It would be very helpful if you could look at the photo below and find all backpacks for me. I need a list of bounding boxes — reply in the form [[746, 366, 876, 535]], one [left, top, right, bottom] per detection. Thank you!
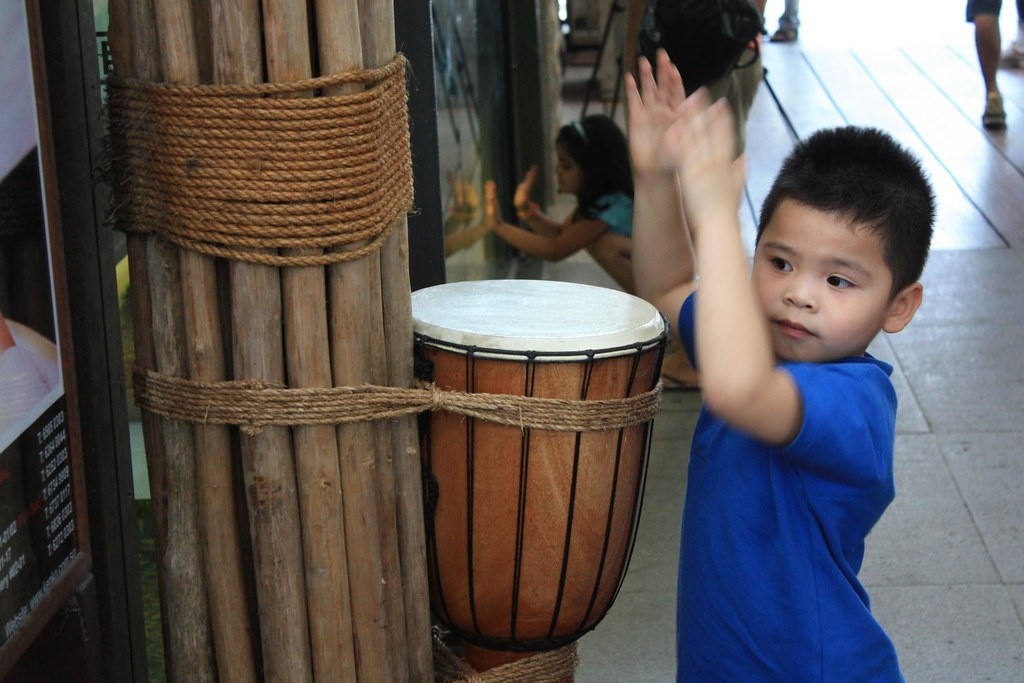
[[638, 0, 768, 97]]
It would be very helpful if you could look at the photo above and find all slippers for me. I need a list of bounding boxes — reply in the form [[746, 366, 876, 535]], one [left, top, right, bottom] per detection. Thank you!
[[660, 372, 700, 393]]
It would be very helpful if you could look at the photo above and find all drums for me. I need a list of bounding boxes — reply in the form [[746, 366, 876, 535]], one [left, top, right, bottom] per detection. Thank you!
[[415, 276, 670, 683]]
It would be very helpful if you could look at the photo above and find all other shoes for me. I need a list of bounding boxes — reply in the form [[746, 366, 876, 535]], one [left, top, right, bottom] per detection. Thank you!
[[998, 41, 1024, 70], [982, 91, 1007, 128], [770, 28, 798, 42]]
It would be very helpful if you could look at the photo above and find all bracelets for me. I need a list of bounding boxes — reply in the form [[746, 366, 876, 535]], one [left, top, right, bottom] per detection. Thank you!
[[517, 201, 538, 221]]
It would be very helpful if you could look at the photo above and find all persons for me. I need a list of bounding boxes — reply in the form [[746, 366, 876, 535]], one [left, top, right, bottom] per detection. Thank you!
[[755, 0, 800, 42], [486, 114, 636, 295], [443, 170, 486, 258], [639, 0, 764, 390], [966, 0, 1024, 127], [625, 49, 937, 683]]
